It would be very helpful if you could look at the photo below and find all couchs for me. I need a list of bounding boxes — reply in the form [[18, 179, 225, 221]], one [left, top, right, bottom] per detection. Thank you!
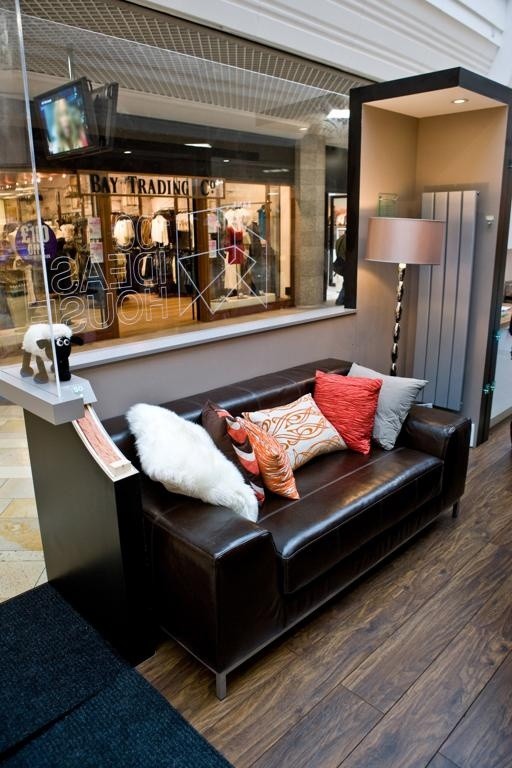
[[100, 355, 472, 700]]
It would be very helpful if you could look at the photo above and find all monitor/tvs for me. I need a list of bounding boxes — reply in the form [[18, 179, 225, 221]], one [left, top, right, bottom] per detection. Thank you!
[[91, 81, 120, 153], [32, 73, 103, 162]]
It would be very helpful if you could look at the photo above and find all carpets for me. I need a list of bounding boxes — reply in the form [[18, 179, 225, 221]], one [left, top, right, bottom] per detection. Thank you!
[[1, 584, 236, 767]]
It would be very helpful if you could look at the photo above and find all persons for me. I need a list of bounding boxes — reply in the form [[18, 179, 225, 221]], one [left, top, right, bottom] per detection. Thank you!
[[334, 229, 347, 304], [50, 98, 85, 152], [225, 214, 246, 265]]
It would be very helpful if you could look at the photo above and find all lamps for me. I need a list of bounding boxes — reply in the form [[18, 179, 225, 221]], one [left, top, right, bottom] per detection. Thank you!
[[363, 217, 448, 376]]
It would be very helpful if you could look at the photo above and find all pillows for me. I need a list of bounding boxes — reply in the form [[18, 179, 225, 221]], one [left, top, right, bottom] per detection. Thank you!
[[309, 367, 385, 457], [125, 400, 264, 524], [238, 391, 350, 474], [346, 361, 429, 454], [188, 397, 267, 507], [233, 414, 302, 502]]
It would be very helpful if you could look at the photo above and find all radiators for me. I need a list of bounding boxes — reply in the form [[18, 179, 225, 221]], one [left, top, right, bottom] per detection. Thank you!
[[412, 189, 479, 413]]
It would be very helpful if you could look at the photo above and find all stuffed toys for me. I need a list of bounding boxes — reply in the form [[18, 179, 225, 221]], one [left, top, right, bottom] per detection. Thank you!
[[18, 322, 87, 385]]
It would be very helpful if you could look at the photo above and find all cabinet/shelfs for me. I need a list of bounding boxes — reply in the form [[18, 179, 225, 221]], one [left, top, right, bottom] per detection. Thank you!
[[489, 326, 512, 430]]
